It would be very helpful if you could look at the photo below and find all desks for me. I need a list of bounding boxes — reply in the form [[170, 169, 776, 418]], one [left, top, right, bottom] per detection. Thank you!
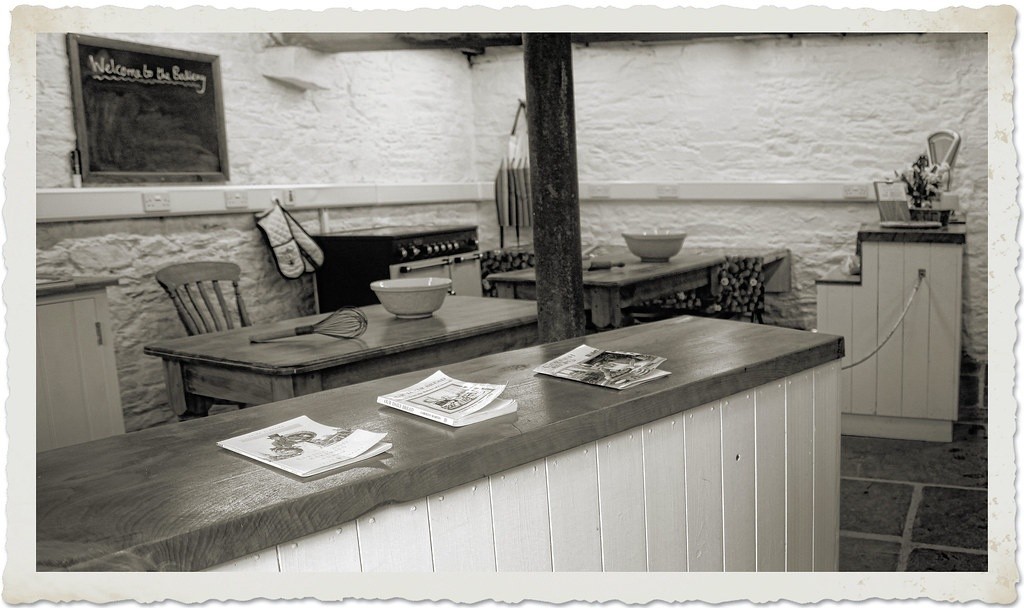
[[144, 296, 538, 422], [486, 247, 792, 341]]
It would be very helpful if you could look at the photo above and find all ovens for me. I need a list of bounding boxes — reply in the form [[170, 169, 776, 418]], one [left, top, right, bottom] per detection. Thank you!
[[310, 224, 484, 311]]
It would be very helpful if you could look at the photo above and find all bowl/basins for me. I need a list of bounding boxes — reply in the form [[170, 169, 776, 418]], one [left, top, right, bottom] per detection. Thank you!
[[619, 232, 687, 263], [370, 277, 452, 319]]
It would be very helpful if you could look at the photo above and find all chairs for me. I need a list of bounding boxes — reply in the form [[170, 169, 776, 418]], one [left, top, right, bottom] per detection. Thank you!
[[155, 262, 252, 338]]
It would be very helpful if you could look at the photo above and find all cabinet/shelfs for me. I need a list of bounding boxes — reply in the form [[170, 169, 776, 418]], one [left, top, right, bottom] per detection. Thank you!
[[36, 273, 125, 453], [816, 218, 968, 443]]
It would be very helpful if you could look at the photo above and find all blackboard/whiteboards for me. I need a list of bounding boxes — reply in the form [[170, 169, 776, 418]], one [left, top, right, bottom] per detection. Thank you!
[[65, 32, 232, 184]]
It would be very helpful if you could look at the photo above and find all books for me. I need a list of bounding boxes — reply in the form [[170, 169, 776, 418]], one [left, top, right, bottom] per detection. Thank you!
[[533, 345, 671, 390], [215, 415, 393, 478], [377, 370, 518, 427]]
